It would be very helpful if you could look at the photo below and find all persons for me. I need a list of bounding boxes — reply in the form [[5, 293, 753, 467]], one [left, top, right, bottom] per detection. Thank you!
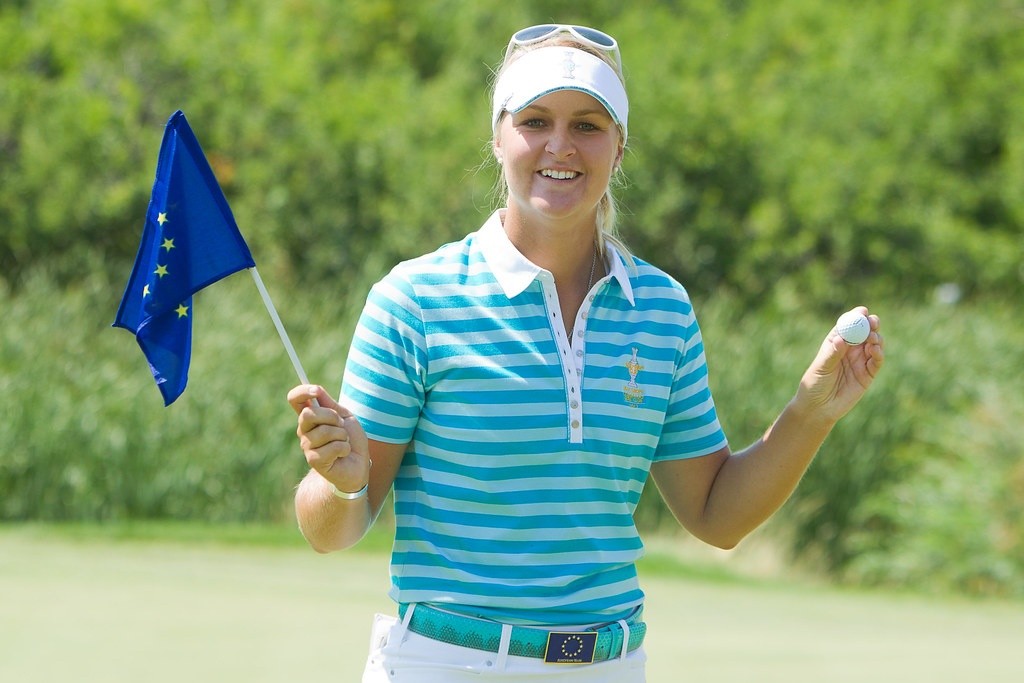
[[288, 25, 884, 683]]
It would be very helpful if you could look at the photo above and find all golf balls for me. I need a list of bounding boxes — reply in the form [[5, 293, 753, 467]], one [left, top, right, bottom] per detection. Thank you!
[[836, 311, 871, 345]]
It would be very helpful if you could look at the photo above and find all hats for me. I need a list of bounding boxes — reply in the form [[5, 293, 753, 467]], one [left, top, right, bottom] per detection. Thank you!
[[491, 46, 629, 148]]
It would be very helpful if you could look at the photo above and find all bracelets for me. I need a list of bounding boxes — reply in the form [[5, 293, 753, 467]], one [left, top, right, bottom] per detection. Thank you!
[[329, 483, 369, 500]]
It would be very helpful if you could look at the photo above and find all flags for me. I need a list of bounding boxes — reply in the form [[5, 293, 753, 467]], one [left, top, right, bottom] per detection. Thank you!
[[111, 111, 255, 407]]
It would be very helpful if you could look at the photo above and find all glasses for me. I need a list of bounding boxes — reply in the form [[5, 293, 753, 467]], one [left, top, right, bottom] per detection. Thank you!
[[502, 24, 622, 76]]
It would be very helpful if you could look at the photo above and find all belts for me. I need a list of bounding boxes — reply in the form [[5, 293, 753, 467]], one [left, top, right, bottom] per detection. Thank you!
[[398, 602, 647, 665]]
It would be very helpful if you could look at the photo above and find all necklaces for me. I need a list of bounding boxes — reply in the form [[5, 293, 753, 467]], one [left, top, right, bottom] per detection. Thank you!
[[567, 242, 596, 341]]
